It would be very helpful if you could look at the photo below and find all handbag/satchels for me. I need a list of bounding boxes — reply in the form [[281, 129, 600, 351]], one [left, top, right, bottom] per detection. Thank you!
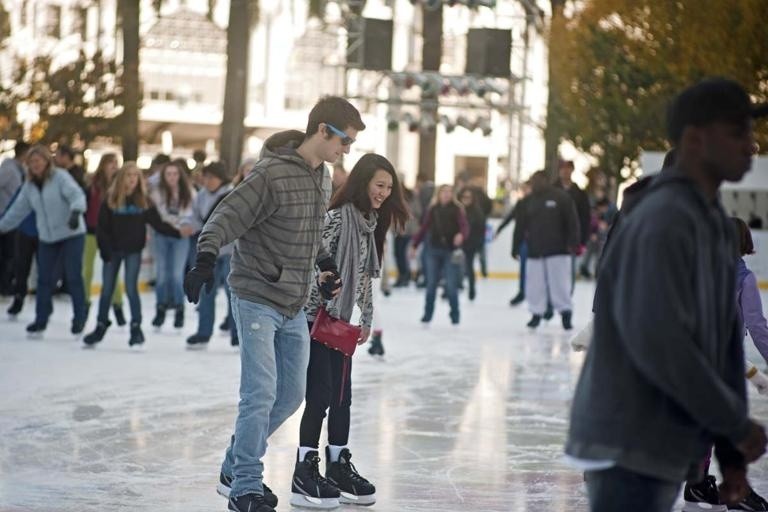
[[311, 306, 360, 356]]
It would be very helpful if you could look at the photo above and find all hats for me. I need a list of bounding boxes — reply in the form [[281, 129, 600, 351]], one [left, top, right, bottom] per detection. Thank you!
[[667, 79, 768, 122]]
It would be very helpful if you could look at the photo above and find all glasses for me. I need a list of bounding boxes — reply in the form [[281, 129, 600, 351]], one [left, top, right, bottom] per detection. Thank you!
[[327, 124, 353, 145]]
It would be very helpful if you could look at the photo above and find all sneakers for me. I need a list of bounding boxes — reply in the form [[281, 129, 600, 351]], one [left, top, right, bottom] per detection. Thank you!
[[7, 296, 209, 346], [220, 446, 375, 512], [685, 475, 768, 512]]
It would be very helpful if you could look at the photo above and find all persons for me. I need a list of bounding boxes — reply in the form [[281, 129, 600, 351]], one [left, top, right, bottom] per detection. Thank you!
[[332, 163, 349, 199], [290, 153, 413, 499], [683, 217, 768, 512], [368, 161, 616, 354], [562, 78, 768, 512], [0, 142, 258, 346], [184, 98, 367, 512]]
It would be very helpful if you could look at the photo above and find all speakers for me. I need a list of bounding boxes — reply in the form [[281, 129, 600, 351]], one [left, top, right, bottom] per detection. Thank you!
[[347, 17, 393, 71], [465, 28, 512, 78]]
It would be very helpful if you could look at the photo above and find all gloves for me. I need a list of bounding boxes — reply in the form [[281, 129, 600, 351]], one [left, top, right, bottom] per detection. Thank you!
[[318, 258, 342, 299], [185, 252, 214, 304]]
